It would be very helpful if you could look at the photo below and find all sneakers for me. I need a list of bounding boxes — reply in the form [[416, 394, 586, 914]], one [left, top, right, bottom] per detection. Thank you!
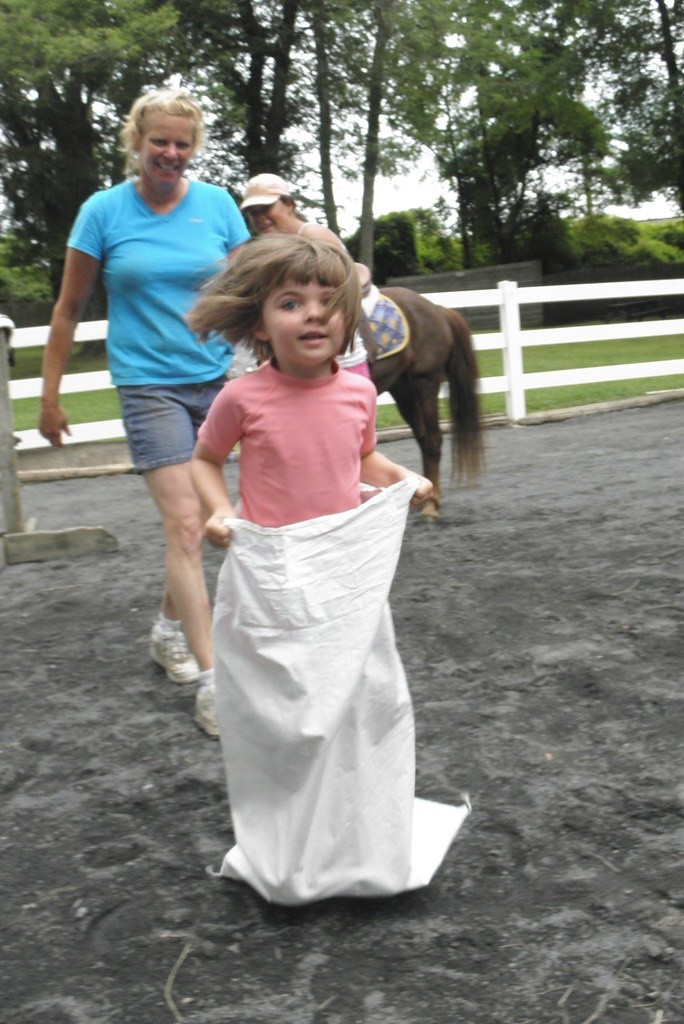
[[194, 683, 223, 740], [149, 622, 202, 684]]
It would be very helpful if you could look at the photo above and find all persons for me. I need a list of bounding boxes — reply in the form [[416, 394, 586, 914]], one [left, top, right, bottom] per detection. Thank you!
[[187, 230, 470, 903], [36, 89, 250, 735], [238, 174, 384, 504]]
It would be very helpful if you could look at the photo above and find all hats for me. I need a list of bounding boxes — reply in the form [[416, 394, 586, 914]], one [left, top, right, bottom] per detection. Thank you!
[[240, 173, 291, 210]]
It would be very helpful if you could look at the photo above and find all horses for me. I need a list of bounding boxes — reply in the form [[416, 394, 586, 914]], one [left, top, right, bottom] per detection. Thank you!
[[355, 286, 486, 529]]
